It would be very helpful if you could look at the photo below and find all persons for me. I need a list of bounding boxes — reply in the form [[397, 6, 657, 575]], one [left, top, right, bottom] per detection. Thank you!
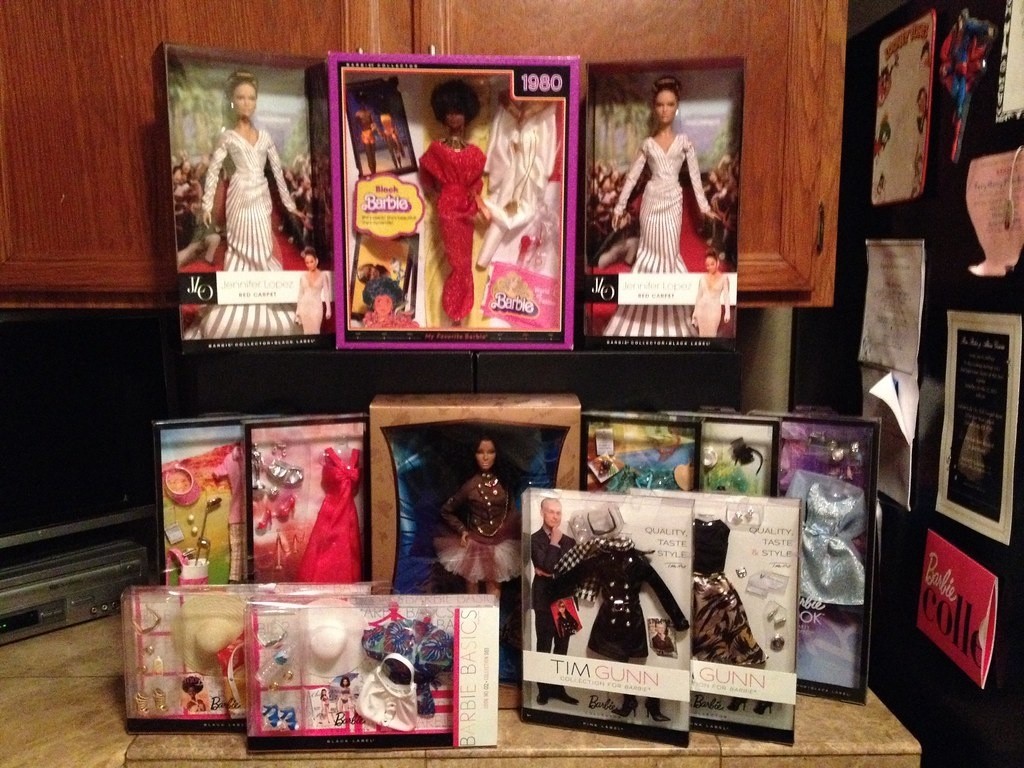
[[374, 100, 406, 172], [531, 497, 582, 705], [603, 73, 722, 336], [433, 434, 522, 606], [417, 77, 491, 329], [318, 689, 331, 725], [691, 248, 732, 339], [338, 676, 353, 713], [182, 676, 207, 713], [350, 95, 383, 175], [295, 245, 332, 336], [555, 600, 578, 638], [653, 621, 676, 653], [186, 65, 309, 339]]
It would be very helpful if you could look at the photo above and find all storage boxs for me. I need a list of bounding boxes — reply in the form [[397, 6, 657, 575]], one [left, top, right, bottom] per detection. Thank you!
[[120, 39, 886, 750]]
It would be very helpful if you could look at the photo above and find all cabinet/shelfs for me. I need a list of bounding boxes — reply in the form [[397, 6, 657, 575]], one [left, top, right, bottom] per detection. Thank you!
[[0, 0, 850, 309]]
